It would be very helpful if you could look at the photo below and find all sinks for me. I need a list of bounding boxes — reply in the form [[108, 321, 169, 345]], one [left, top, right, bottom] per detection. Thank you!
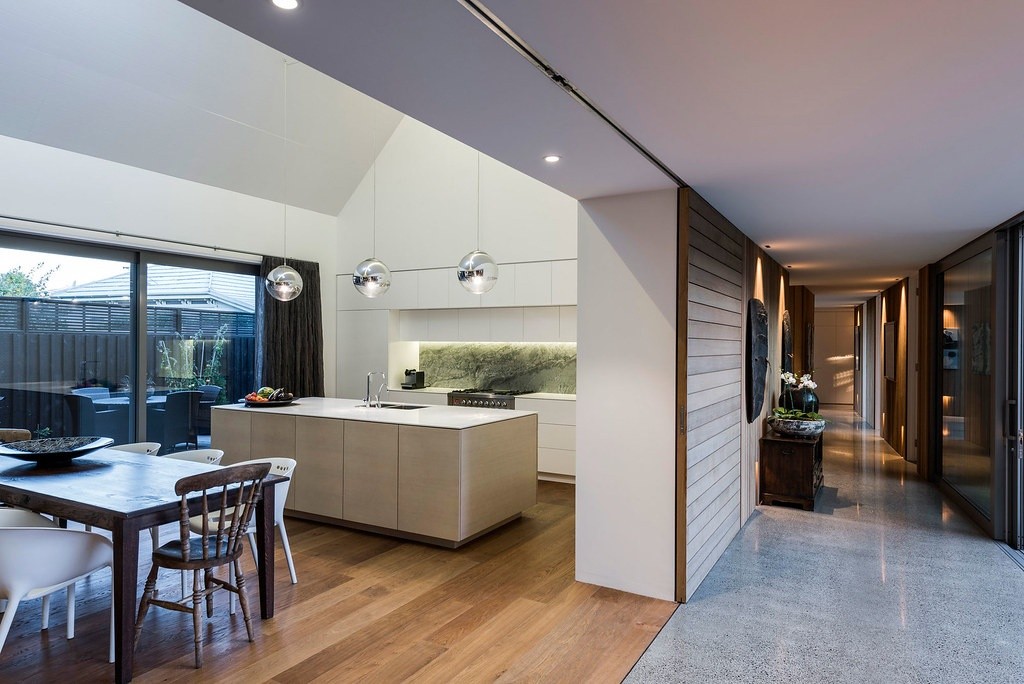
[[390, 404, 429, 410], [354, 403, 393, 409]]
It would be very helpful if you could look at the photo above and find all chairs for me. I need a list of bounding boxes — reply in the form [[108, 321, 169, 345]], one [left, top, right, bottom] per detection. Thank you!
[[72, 388, 110, 411], [147, 391, 204, 454], [64, 394, 129, 446], [0, 444, 297, 669], [196, 386, 222, 434]]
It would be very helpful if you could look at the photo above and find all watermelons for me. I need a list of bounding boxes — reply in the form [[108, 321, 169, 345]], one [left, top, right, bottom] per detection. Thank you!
[[258, 386, 275, 398]]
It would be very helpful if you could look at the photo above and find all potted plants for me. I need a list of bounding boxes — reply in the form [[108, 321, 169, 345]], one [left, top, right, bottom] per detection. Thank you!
[[767, 407, 832, 440]]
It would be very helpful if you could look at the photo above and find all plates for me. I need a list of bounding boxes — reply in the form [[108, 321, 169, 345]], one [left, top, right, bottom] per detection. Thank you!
[[0, 436, 114, 468], [238, 397, 299, 405]]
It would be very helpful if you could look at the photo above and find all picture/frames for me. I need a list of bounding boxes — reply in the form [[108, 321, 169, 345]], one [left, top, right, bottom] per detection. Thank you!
[[883, 321, 896, 381]]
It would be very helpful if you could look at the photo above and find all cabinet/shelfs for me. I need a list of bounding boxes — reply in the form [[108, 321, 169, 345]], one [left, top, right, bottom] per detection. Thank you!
[[514, 398, 577, 477], [336, 260, 578, 309], [759, 430, 825, 512]]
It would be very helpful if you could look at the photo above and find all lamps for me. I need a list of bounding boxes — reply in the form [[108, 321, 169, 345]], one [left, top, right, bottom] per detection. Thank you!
[[266, 60, 303, 302], [352, 132, 390, 297], [457, 153, 498, 295]]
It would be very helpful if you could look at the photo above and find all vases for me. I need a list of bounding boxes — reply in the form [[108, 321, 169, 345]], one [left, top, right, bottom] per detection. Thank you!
[[778, 383, 819, 417]]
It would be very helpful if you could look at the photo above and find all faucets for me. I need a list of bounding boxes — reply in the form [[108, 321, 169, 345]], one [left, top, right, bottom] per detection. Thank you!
[[363, 371, 389, 408]]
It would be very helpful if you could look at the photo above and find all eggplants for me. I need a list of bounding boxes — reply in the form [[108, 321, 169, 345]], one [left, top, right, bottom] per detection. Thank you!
[[268, 387, 294, 399]]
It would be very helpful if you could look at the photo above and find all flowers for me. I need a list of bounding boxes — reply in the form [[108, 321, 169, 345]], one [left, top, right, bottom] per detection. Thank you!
[[772, 364, 832, 424]]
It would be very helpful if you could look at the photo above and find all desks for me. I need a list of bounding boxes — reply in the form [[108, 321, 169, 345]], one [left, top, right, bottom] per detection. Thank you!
[[0, 449, 291, 684], [92, 396, 167, 409]]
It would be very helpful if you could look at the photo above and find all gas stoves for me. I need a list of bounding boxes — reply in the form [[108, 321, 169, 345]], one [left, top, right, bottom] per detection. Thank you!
[[448, 387, 534, 409]]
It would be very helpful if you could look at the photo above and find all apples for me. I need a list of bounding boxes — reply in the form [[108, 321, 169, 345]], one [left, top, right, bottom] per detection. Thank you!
[[245, 392, 270, 402]]
